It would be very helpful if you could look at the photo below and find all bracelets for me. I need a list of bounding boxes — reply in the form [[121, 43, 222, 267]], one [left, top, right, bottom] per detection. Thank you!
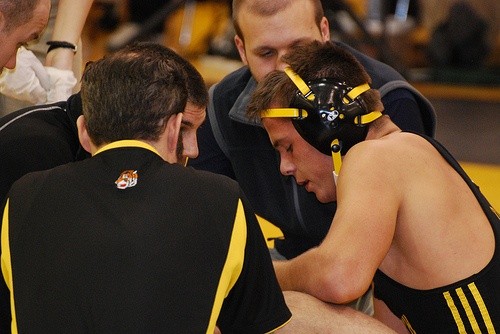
[[45, 40, 77, 52]]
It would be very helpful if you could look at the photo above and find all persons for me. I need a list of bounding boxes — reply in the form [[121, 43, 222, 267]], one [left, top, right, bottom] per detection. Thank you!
[[1, 45, 209, 199], [187, 0, 438, 259], [1, 1, 50, 72], [0, 46, 292, 334], [1, 0, 93, 105], [244, 39, 500, 334]]
